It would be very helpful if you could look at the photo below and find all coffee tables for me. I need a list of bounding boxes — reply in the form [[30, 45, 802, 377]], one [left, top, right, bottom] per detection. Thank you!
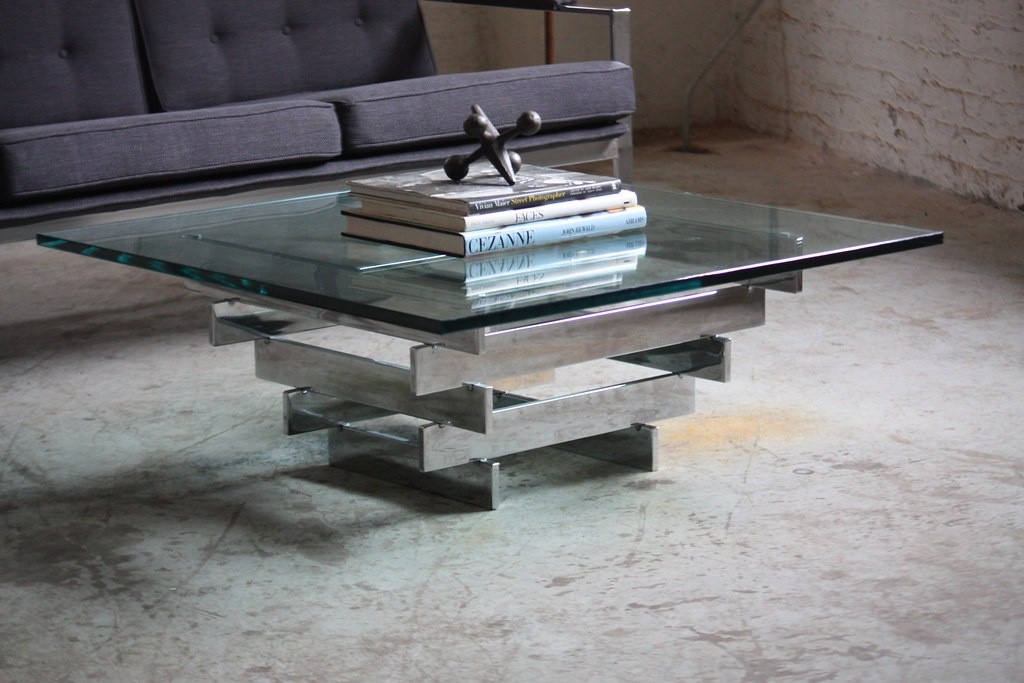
[[38, 180, 945, 511]]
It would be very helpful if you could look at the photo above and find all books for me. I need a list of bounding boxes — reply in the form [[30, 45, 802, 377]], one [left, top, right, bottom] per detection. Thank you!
[[343, 233, 646, 310], [340, 164, 646, 258]]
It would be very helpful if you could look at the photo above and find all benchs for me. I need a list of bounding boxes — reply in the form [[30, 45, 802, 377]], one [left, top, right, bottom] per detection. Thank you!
[[0, 0, 636, 245]]
